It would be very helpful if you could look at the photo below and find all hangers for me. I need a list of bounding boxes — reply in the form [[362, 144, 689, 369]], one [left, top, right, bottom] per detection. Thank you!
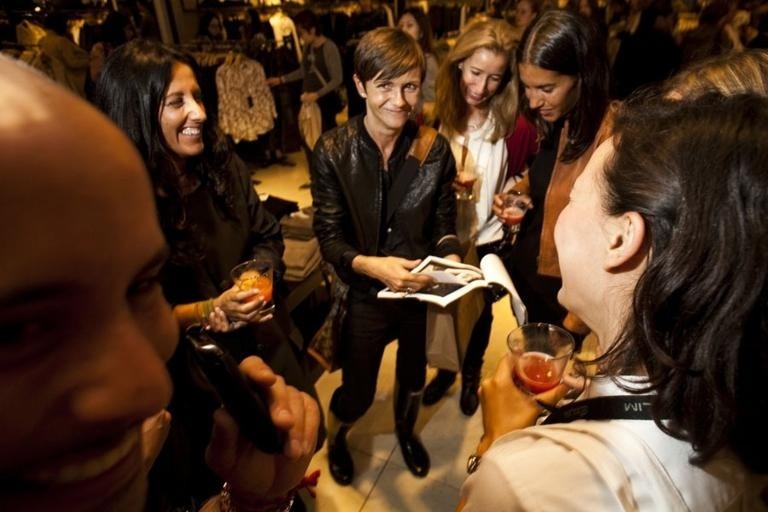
[[195, 46, 242, 67]]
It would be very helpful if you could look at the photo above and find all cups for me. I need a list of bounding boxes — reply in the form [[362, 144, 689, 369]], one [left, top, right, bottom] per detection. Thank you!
[[453, 169, 479, 201], [232, 260, 273, 312], [506, 325, 575, 392], [501, 192, 527, 226]]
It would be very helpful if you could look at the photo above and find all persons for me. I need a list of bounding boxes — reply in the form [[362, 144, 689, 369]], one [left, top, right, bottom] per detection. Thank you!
[[0, 2, 768, 512]]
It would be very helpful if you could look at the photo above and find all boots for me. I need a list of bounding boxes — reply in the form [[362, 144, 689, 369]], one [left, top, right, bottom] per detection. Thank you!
[[459, 360, 481, 417], [393, 379, 431, 479], [326, 411, 355, 486], [422, 367, 457, 406]]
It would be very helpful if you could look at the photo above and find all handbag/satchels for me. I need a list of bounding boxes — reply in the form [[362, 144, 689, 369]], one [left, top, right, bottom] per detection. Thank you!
[[304, 287, 347, 373]]
